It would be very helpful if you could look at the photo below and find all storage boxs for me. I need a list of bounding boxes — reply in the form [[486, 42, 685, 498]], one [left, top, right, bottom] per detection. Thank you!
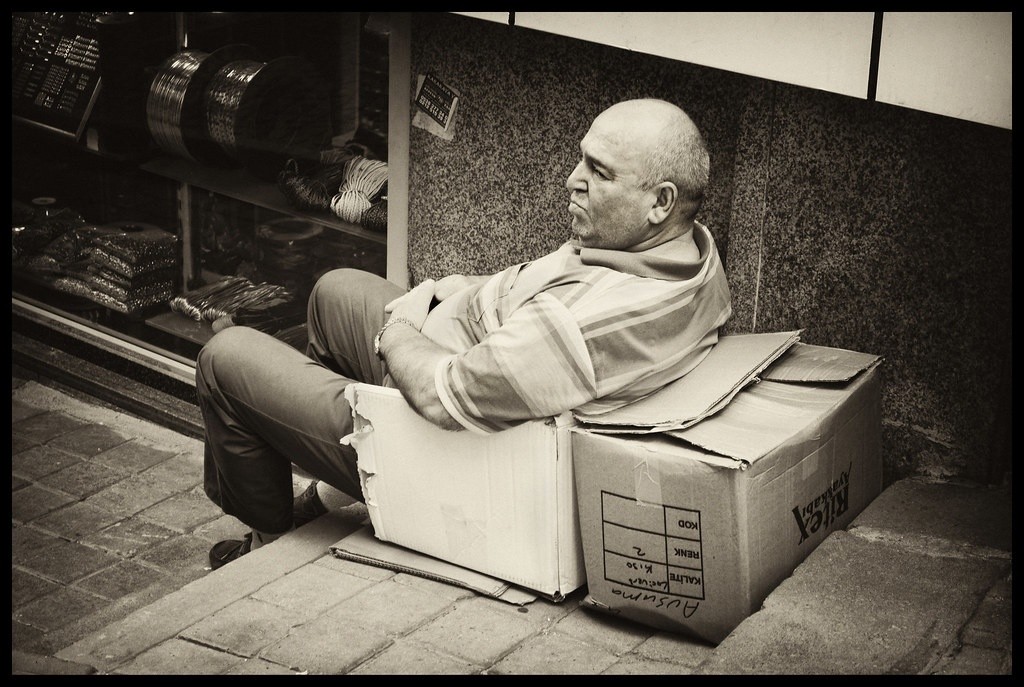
[[570, 332, 886, 646], [341, 380, 587, 606]]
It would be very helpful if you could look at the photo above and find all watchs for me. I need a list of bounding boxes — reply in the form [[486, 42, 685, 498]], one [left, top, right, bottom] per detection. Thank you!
[[373, 317, 418, 359]]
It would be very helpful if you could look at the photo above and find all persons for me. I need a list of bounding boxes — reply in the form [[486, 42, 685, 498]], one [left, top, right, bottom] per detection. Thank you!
[[193, 99, 731, 571]]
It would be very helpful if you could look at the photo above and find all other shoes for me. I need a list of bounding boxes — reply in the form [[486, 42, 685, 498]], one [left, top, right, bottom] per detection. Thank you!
[[293, 481, 329, 528], [209, 532, 253, 571]]
